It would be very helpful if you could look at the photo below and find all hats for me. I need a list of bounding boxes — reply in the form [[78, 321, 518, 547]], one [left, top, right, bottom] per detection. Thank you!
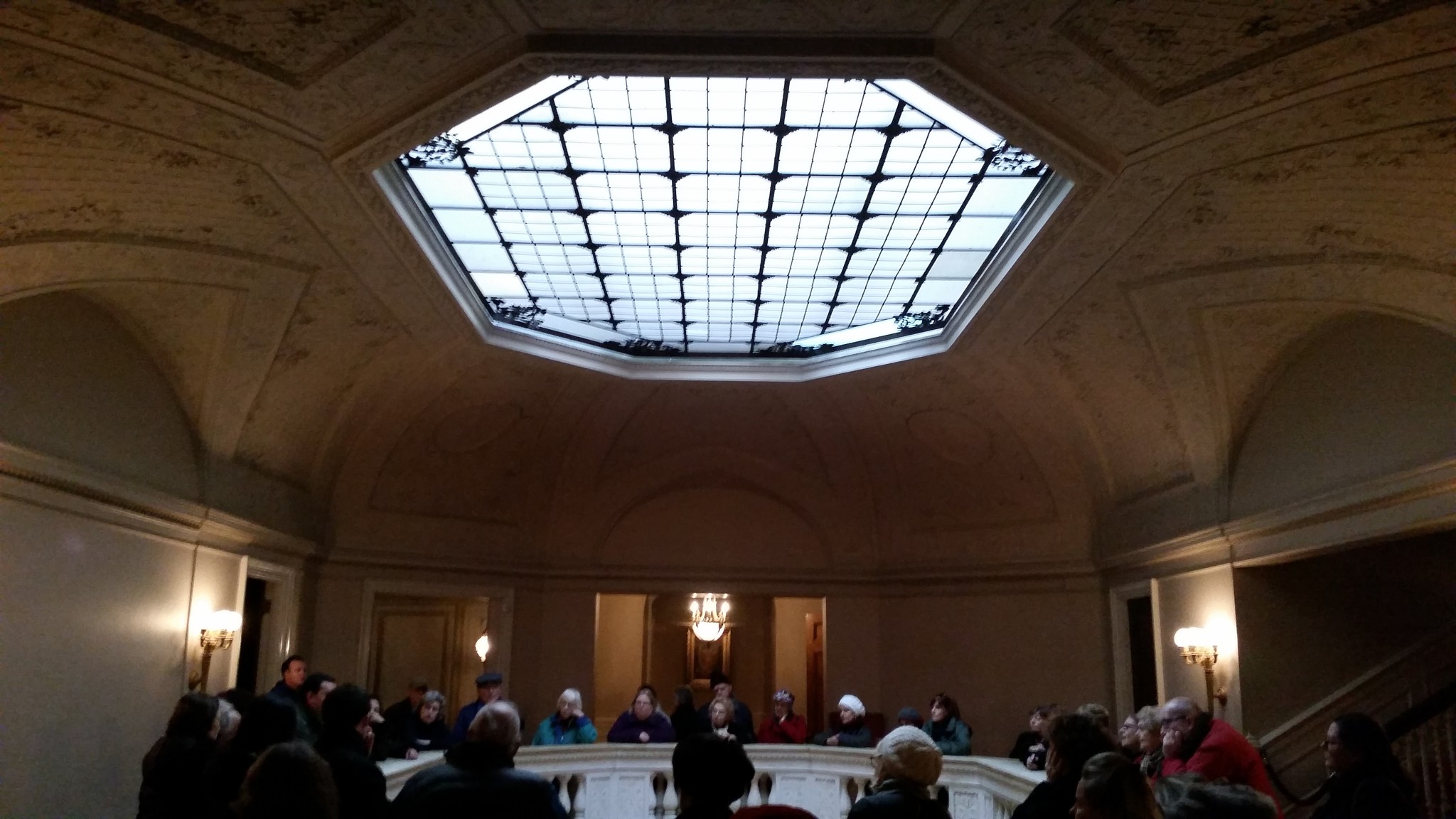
[[773, 687, 795, 703], [674, 735, 754, 801], [839, 693, 866, 718], [475, 672, 503, 685], [709, 668, 731, 689], [876, 724, 944, 784], [318, 684, 371, 734]]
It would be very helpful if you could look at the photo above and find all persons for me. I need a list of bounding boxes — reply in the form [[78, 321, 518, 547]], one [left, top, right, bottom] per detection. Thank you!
[[607, 689, 677, 744], [697, 696, 739, 744], [447, 672, 525, 751], [696, 674, 758, 744], [531, 688, 598, 747], [757, 689, 808, 744], [921, 692, 972, 756], [627, 683, 671, 723], [136, 649, 444, 818], [668, 684, 698, 729], [1009, 685, 1283, 819], [1305, 713, 1430, 819], [670, 730, 756, 819], [815, 693, 877, 748], [391, 701, 571, 819], [896, 706, 925, 730], [845, 725, 954, 819]]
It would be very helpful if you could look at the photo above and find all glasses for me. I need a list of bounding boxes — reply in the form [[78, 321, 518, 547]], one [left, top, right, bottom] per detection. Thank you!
[[869, 756, 880, 769], [1323, 736, 1341, 746], [1161, 716, 1185, 729], [1122, 723, 1136, 729]]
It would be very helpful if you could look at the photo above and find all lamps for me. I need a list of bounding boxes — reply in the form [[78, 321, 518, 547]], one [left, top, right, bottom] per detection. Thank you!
[[188, 606, 243, 687], [1173, 624, 1228, 720], [685, 593, 733, 645]]
